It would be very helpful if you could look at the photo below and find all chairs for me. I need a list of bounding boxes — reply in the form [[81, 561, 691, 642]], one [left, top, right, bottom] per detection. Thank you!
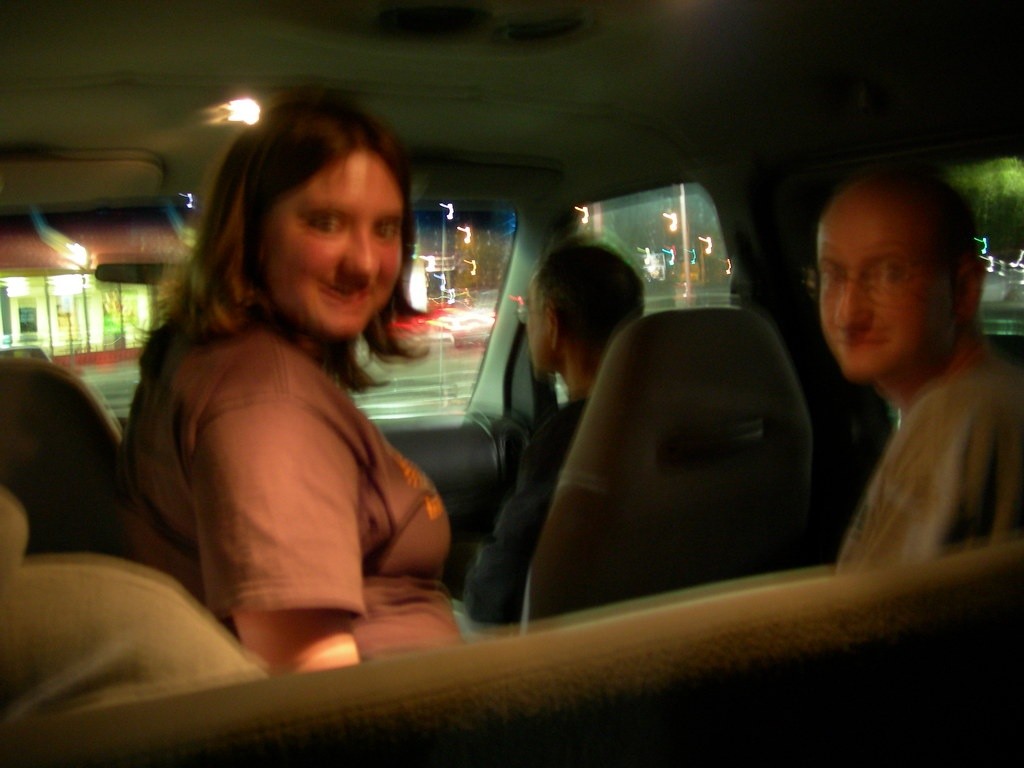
[[520, 306, 815, 637]]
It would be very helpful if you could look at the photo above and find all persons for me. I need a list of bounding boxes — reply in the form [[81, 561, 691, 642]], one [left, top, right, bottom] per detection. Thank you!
[[0, 480, 269, 731], [463, 236, 644, 624], [815, 170, 1024, 571], [110, 89, 462, 679]]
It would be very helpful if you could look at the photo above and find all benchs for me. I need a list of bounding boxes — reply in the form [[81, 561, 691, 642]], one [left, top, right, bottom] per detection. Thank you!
[[0, 542, 1024, 768]]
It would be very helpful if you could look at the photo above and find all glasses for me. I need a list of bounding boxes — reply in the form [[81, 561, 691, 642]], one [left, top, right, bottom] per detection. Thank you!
[[802, 262, 929, 297], [514, 304, 543, 324]]
